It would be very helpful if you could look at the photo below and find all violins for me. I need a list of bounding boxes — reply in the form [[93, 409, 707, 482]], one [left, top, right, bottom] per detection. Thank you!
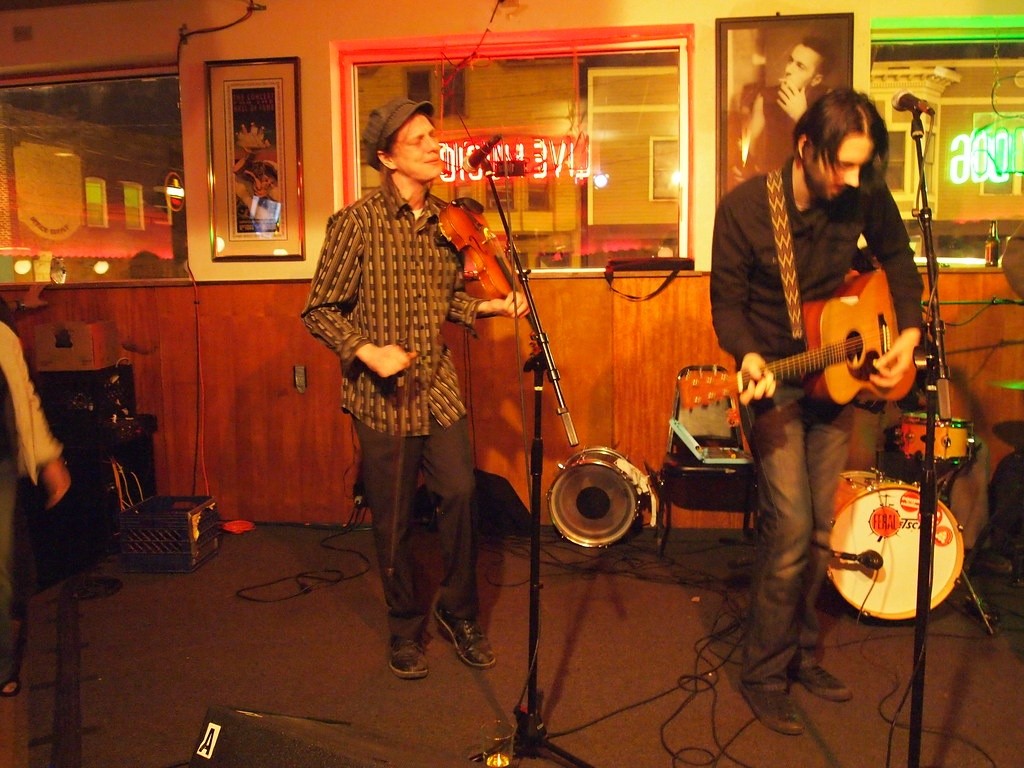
[[439, 195, 543, 359]]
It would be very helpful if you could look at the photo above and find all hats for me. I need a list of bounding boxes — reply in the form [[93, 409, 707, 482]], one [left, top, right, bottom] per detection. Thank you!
[[363, 98, 435, 172]]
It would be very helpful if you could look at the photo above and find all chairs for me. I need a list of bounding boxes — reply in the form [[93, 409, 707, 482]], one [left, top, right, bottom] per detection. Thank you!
[[656, 363, 759, 557]]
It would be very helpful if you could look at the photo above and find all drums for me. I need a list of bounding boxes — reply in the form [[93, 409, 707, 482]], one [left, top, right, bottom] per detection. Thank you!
[[549, 447, 639, 549], [827, 467, 967, 622], [897, 412, 975, 465]]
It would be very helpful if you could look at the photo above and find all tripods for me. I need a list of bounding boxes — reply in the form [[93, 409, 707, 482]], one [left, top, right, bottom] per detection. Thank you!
[[469, 357, 596, 768]]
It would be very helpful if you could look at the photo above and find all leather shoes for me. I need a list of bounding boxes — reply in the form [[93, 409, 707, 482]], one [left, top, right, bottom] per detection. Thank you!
[[434, 607, 496, 670], [389, 635, 430, 679]]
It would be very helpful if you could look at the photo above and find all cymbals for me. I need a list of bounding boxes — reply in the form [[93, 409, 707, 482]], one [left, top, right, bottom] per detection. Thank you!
[[986, 378, 1024, 391]]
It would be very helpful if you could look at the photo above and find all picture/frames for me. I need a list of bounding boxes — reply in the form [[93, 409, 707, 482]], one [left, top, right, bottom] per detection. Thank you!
[[714, 11, 855, 209], [647, 134, 679, 202], [203, 56, 306, 262]]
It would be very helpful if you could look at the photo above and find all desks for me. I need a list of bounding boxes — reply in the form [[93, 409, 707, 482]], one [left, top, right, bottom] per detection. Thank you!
[[18, 433, 154, 557]]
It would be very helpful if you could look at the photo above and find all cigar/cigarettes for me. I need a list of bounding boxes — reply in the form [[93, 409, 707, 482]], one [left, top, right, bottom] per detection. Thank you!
[[778, 79, 786, 83]]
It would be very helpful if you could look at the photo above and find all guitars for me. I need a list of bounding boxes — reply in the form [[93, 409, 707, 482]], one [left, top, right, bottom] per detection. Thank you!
[[677, 270, 917, 411]]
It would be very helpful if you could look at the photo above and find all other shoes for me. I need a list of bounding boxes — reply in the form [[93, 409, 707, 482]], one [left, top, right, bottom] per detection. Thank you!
[[786, 663, 852, 702], [0, 673, 21, 696], [739, 683, 805, 735]]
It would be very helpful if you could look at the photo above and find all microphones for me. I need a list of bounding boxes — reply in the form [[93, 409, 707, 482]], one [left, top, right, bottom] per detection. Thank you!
[[891, 89, 935, 117], [462, 133, 503, 173]]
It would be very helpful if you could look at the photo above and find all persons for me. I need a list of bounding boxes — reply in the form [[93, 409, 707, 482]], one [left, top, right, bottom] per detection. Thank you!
[[943, 436, 1019, 574], [726, 36, 837, 204], [710, 87, 929, 737], [302, 98, 531, 680], [0, 319, 71, 697]]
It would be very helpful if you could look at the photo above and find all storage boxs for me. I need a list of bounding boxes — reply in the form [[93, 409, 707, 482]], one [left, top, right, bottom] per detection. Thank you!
[[36, 318, 118, 371], [119, 495, 222, 573]]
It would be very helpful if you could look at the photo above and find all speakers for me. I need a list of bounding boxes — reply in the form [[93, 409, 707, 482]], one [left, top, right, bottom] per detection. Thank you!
[[189, 704, 487, 768]]
